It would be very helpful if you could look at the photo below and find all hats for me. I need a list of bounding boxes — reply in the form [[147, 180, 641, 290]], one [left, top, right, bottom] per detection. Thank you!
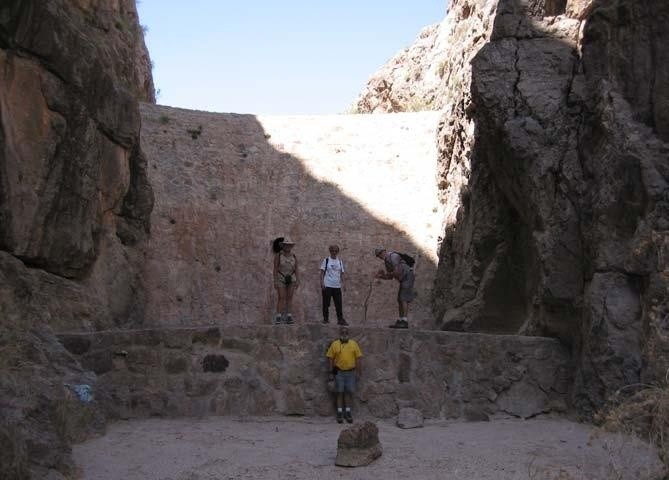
[[279, 235, 296, 247], [375, 246, 385, 257]]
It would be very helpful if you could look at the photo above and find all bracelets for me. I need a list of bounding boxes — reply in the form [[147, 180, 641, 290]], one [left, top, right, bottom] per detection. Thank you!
[[329, 371, 333, 374]]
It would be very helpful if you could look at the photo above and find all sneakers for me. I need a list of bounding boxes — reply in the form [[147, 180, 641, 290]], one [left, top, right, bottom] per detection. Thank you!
[[276, 316, 281, 324], [345, 411, 352, 423], [337, 412, 343, 423], [389, 320, 400, 328], [287, 316, 293, 323], [337, 318, 349, 325], [323, 319, 329, 323], [397, 320, 408, 329]]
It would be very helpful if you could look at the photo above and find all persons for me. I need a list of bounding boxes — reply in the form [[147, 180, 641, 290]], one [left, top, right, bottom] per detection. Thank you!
[[327, 326, 363, 424], [373, 246, 414, 330], [274, 235, 300, 323], [318, 243, 349, 326]]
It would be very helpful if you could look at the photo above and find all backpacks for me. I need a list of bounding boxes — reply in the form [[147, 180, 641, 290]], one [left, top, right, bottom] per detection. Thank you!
[[390, 250, 415, 268]]
